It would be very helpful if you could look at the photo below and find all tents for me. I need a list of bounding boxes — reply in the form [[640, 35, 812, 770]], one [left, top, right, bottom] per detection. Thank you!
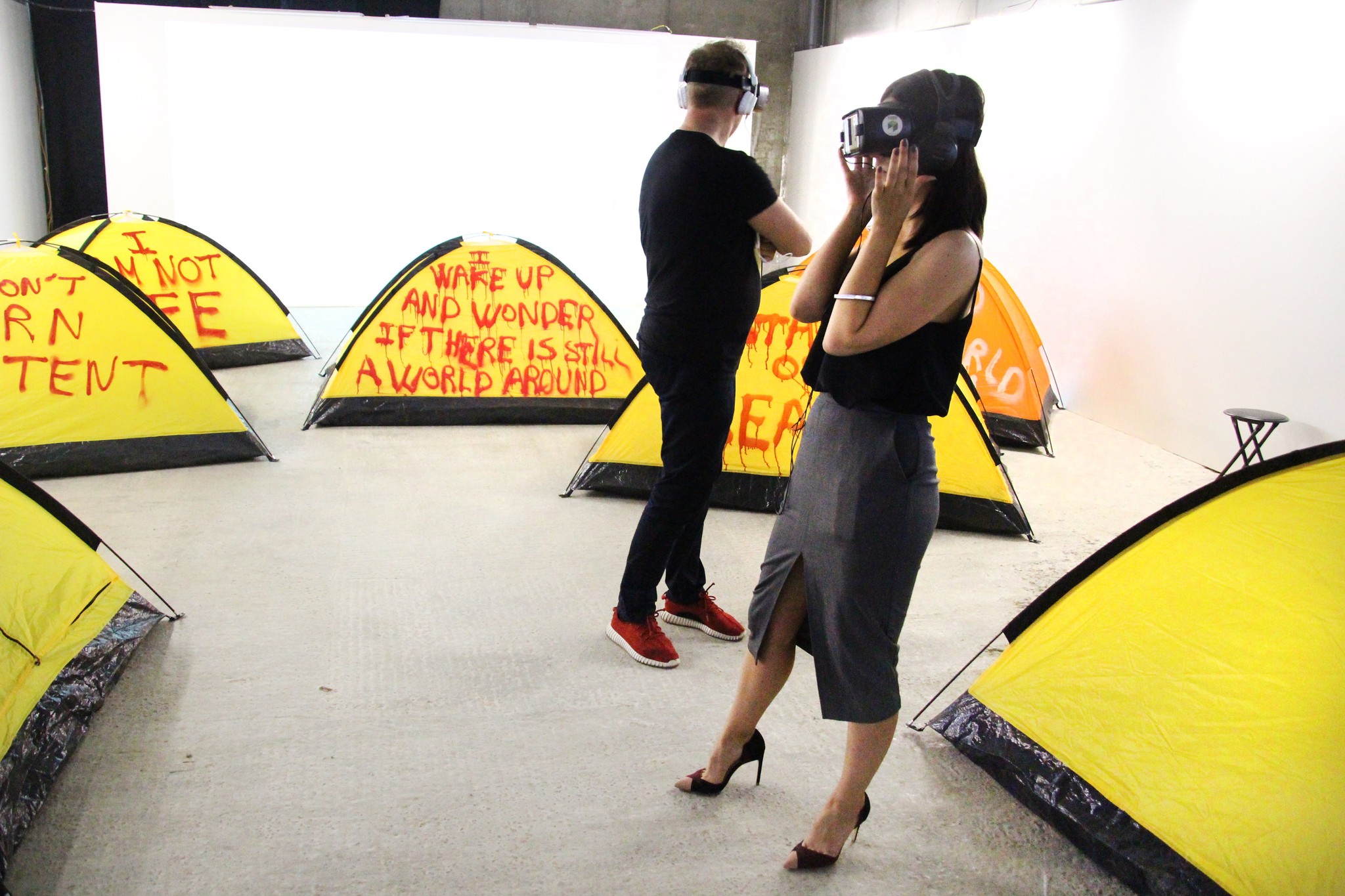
[[908, 440, 1345, 896], [301, 233, 647, 431], [562, 265, 1040, 543], [787, 225, 1064, 458], [29, 211, 322, 371], [0, 460, 186, 896], [0, 239, 275, 479]]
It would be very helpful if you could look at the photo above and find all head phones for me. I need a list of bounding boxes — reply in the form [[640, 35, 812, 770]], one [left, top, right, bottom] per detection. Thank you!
[[914, 70, 961, 171], [678, 40, 758, 114]]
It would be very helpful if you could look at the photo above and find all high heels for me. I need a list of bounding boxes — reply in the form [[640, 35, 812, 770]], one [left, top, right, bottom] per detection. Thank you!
[[783, 792, 870, 869], [673, 728, 765, 794]]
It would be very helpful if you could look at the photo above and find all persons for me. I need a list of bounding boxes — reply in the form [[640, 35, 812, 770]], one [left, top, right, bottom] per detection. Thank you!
[[673, 69, 987, 870], [606, 41, 812, 668]]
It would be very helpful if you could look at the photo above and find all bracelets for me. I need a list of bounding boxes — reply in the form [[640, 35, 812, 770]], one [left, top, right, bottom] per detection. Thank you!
[[834, 294, 876, 301]]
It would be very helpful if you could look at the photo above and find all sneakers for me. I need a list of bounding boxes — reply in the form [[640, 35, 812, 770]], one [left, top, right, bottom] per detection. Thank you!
[[605, 607, 680, 668], [660, 583, 746, 641]]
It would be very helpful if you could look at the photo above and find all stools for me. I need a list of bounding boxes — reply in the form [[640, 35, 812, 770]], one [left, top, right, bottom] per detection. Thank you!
[[1212, 407, 1291, 482]]
